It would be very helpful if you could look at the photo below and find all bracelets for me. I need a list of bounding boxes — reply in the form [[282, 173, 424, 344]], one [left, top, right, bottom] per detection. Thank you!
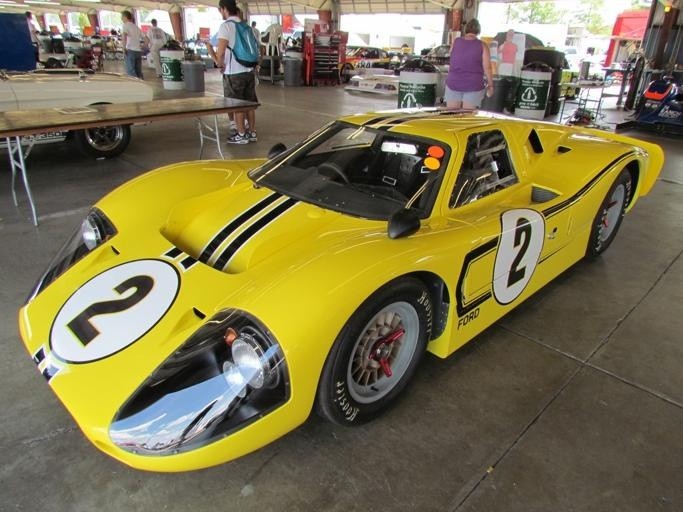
[[487, 86, 493, 88]]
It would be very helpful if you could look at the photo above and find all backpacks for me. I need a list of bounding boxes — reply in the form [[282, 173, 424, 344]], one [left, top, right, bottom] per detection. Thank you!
[[225, 20, 258, 67]]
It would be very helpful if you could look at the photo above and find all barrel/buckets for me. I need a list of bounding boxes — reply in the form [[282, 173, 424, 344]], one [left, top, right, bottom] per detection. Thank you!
[[160, 50, 185, 90], [283, 59, 306, 86], [397, 72, 436, 108], [513, 70, 552, 120]]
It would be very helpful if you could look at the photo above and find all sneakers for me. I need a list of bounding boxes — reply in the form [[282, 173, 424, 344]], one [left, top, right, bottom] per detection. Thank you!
[[227, 119, 257, 144]]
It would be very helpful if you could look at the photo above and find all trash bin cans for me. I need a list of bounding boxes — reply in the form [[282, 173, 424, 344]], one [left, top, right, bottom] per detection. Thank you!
[[284, 57, 303, 87]]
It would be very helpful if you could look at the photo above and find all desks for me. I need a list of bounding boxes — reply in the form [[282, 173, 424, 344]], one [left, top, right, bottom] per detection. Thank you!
[[558, 81, 610, 125], [0, 95, 263, 227], [598, 68, 655, 112]]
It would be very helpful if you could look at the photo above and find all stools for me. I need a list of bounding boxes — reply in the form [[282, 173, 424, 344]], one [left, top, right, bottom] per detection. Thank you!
[[257, 56, 283, 85]]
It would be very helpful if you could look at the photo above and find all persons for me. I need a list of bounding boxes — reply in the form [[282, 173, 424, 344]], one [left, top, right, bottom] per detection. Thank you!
[[442, 17, 493, 110], [25, 11, 41, 61], [217, 0, 260, 145], [120, 10, 149, 80], [497, 29, 518, 76], [145, 18, 168, 78], [207, 7, 266, 136], [251, 21, 260, 39]]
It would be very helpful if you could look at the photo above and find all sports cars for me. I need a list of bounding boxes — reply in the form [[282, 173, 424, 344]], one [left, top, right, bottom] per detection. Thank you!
[[63, 24, 225, 73], [19, 100, 664, 473]]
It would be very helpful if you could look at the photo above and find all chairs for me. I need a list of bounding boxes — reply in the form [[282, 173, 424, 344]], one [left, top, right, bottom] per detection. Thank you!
[[364, 152, 425, 208], [259, 23, 282, 56]]
